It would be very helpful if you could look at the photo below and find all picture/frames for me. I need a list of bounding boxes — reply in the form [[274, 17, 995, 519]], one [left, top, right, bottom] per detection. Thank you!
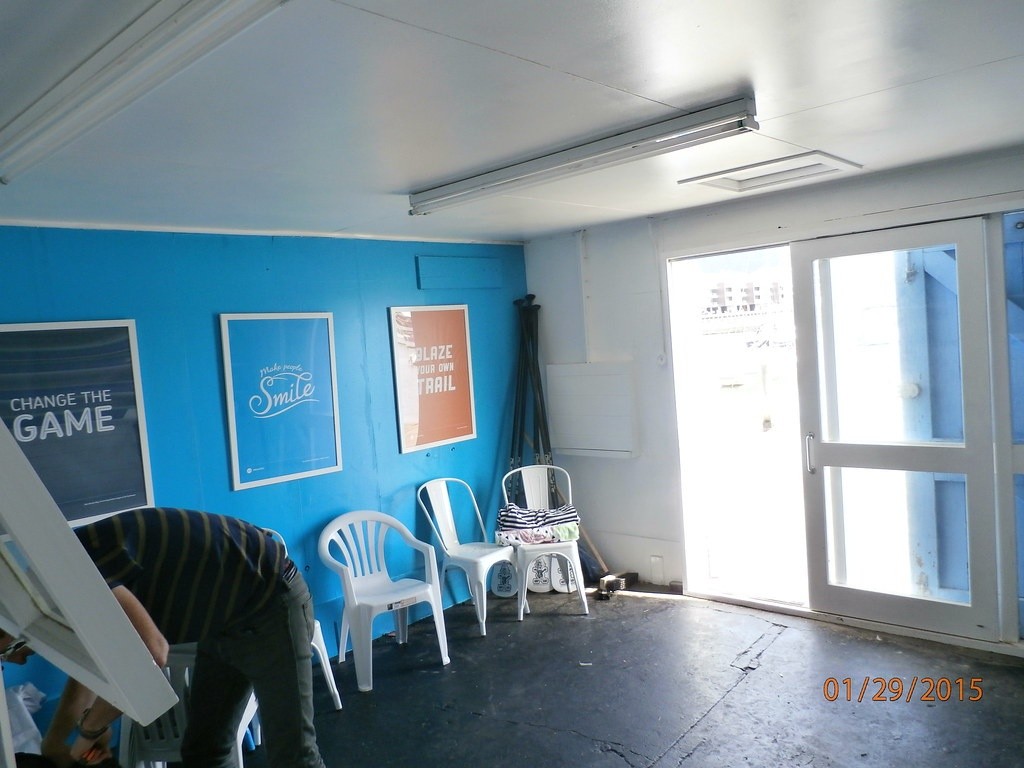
[[1, 319, 155, 542], [219, 312, 344, 493], [390, 304, 477, 454]]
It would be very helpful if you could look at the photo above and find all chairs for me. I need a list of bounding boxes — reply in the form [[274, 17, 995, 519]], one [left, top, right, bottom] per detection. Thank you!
[[416, 478, 530, 636], [317, 510, 451, 691], [502, 464, 589, 621], [120, 528, 344, 768]]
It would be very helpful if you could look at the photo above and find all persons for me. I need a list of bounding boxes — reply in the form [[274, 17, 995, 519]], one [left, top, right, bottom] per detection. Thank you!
[[0, 506, 326, 768]]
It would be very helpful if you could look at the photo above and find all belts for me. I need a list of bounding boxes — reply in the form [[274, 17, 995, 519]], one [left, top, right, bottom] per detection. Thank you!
[[283, 560, 298, 584]]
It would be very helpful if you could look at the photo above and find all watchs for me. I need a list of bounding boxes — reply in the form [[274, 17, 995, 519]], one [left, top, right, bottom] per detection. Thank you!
[[75, 707, 109, 739]]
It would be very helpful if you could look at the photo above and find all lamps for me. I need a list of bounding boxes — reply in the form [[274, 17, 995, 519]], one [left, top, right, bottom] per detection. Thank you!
[[0, 1, 291, 185], [407, 98, 759, 215]]
